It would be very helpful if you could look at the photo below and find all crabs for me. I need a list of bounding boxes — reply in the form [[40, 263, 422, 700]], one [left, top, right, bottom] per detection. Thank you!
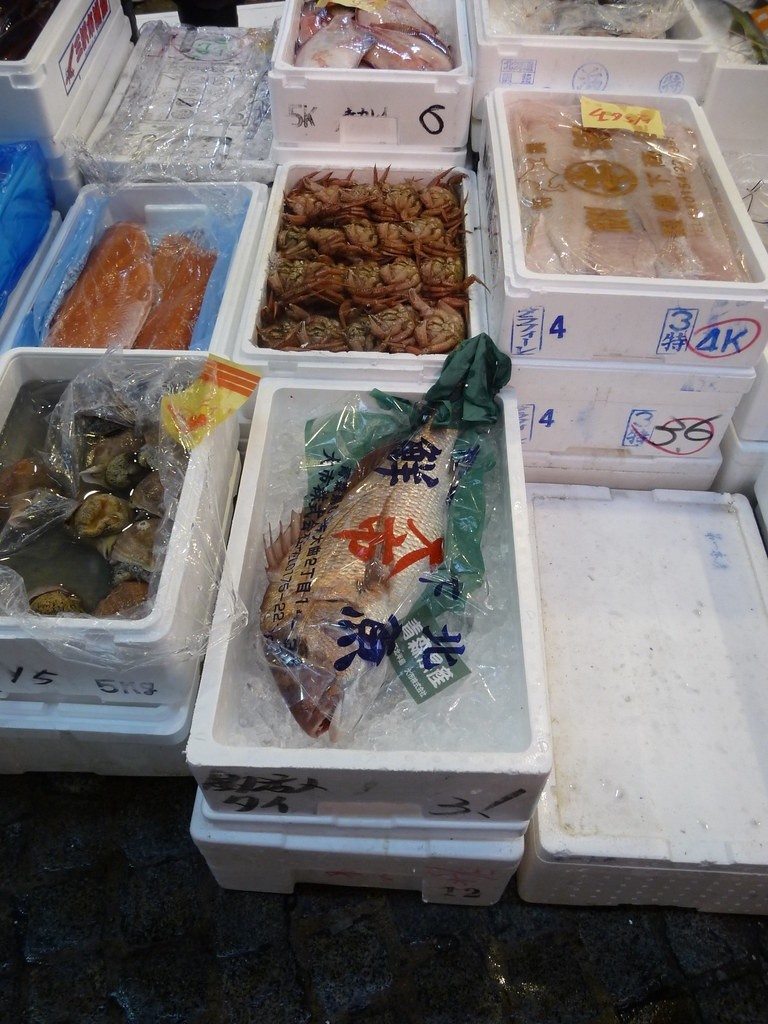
[[254, 162, 494, 355]]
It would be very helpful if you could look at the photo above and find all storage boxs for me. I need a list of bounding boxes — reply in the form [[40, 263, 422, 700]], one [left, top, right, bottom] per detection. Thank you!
[[1, 0, 768, 917]]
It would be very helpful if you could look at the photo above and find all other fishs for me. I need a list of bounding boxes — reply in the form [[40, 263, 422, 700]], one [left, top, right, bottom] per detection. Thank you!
[[260, 391, 460, 736]]
